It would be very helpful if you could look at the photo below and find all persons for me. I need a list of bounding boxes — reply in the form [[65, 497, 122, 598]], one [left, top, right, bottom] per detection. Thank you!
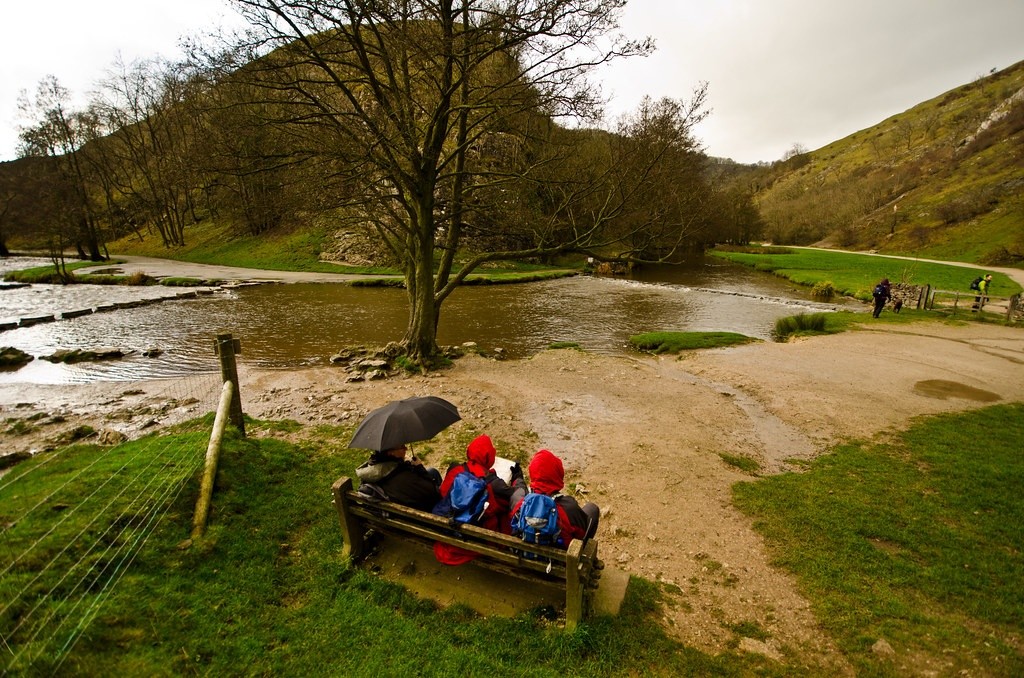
[[440, 435, 530, 546], [511, 450, 600, 552], [356, 444, 442, 512], [972, 274, 992, 312], [872, 279, 892, 318]]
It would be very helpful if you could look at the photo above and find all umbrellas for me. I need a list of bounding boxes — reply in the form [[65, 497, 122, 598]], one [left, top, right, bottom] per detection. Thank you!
[[348, 396, 461, 462]]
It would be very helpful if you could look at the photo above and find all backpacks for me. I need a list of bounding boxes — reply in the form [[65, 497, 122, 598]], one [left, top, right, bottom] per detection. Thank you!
[[872, 283, 887, 298], [433, 462, 499, 523], [510, 486, 565, 573], [970, 277, 988, 291]]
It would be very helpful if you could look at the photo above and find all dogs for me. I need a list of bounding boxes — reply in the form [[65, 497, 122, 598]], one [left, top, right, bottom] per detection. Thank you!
[[893, 299, 902, 314]]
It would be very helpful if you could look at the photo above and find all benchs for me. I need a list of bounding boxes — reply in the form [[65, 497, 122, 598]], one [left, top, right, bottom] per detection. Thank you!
[[332, 476, 605, 634]]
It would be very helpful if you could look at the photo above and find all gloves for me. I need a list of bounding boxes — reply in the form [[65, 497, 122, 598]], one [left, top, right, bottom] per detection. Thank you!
[[986, 298, 989, 302], [510, 463, 523, 482]]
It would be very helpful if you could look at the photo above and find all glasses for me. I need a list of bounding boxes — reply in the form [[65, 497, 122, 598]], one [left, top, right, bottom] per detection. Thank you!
[[395, 446, 407, 451]]
